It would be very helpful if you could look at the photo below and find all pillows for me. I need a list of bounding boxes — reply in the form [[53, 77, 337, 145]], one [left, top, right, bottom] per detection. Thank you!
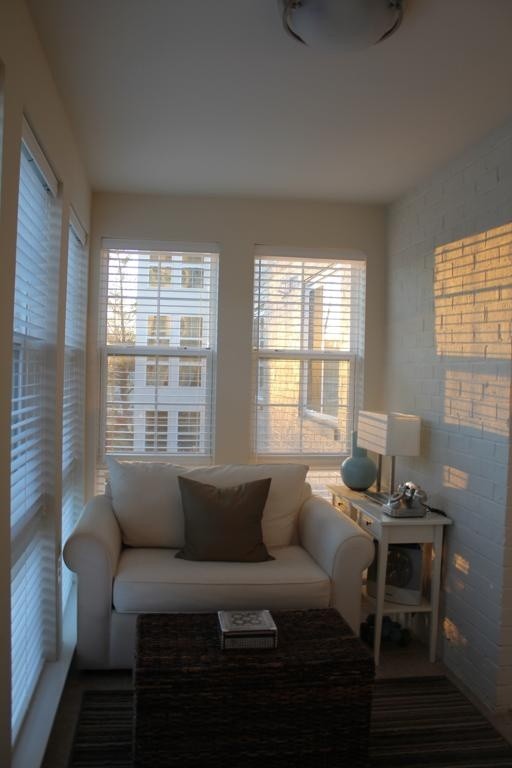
[[174, 475, 274, 564]]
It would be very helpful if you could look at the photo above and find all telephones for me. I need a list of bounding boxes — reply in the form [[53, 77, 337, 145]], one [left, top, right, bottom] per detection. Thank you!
[[381, 481, 427, 518]]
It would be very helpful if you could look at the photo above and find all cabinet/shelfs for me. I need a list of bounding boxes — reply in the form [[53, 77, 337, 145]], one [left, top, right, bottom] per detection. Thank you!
[[328, 484, 452, 668]]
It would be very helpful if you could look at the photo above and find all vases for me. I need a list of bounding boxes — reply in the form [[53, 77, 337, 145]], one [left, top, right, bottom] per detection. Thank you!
[[340, 429, 377, 492]]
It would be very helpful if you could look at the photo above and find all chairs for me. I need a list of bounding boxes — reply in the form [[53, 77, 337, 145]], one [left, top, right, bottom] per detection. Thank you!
[[62, 453, 375, 670]]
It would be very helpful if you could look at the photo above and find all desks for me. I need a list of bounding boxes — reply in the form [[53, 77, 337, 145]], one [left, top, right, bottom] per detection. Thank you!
[[128, 607, 376, 768]]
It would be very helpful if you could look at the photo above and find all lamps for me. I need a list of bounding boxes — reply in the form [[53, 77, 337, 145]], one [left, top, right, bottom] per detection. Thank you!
[[355, 408, 421, 509]]
